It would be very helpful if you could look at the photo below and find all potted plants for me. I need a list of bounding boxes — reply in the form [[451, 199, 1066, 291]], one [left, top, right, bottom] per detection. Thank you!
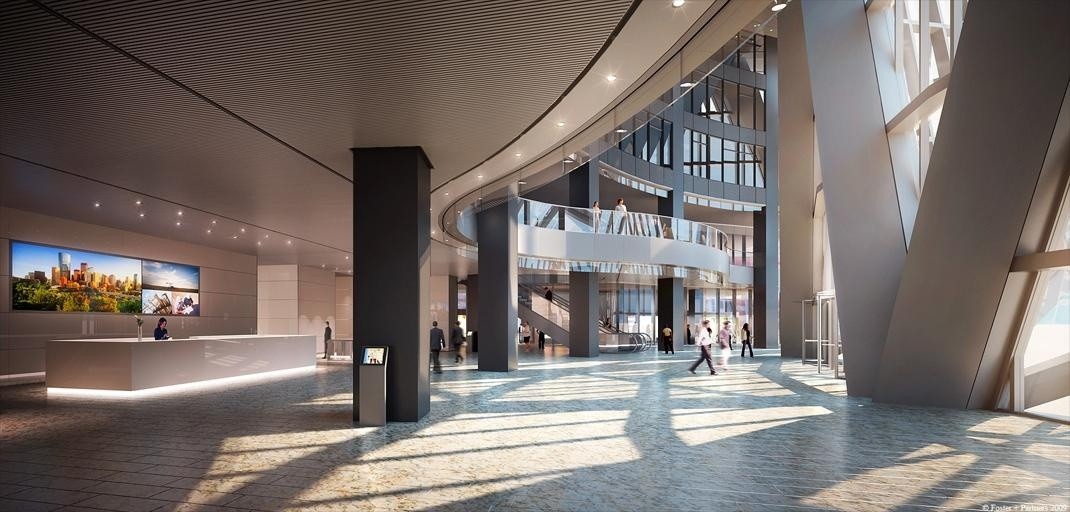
[[134, 316, 144, 342]]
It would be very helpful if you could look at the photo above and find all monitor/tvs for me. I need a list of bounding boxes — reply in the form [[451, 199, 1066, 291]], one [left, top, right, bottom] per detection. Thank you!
[[360, 345, 387, 367]]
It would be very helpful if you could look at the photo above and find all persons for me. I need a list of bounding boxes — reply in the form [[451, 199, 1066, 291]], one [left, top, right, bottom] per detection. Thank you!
[[536, 329, 546, 350], [614, 197, 628, 235], [450, 320, 468, 364], [153, 316, 171, 341], [521, 321, 532, 353], [729, 329, 735, 350], [320, 321, 333, 359], [718, 320, 733, 370], [593, 201, 602, 233], [687, 323, 691, 345], [429, 321, 447, 374], [688, 319, 719, 375], [543, 286, 552, 302], [707, 326, 713, 350], [662, 323, 674, 354], [740, 322, 755, 358], [605, 317, 613, 329]]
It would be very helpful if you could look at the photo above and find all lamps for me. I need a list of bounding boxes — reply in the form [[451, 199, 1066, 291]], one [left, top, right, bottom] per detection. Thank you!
[[769, 1, 789, 12]]
